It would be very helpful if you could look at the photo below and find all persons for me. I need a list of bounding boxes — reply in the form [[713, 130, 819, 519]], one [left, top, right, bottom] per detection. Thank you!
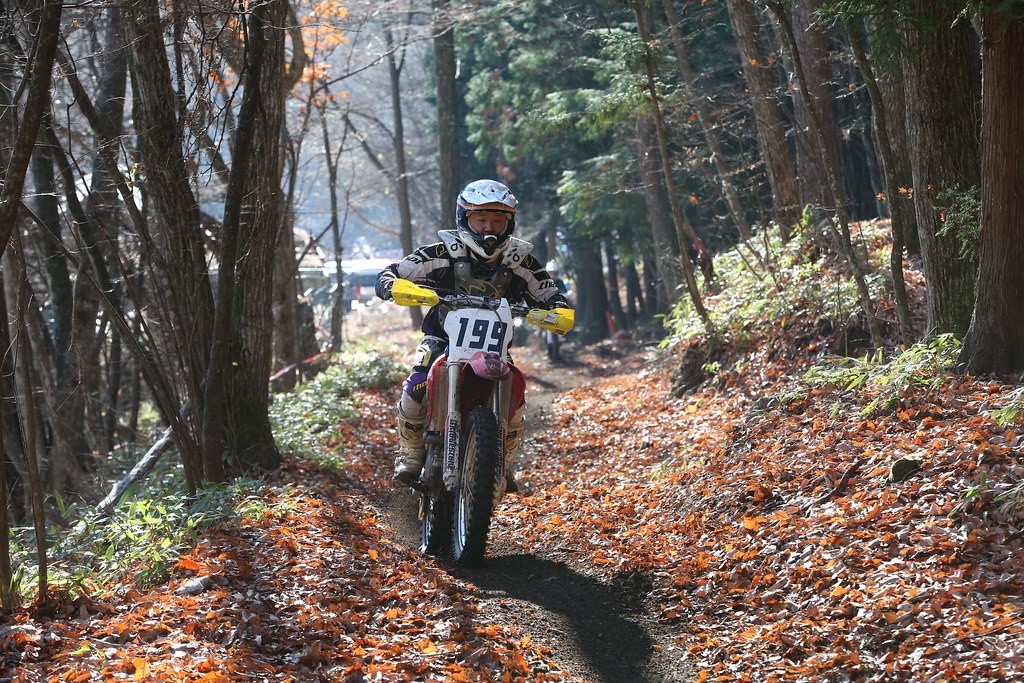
[[550, 271, 566, 294], [376, 179, 572, 493]]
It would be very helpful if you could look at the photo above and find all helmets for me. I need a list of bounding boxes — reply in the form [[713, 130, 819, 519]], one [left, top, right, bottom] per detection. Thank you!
[[455, 179, 518, 258]]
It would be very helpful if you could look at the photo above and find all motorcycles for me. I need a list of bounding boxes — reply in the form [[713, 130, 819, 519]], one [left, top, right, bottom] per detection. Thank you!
[[387, 279, 575, 578]]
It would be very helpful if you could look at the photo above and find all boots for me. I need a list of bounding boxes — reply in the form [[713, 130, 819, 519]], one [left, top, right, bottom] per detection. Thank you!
[[505, 417, 524, 494], [394, 400, 429, 480]]
